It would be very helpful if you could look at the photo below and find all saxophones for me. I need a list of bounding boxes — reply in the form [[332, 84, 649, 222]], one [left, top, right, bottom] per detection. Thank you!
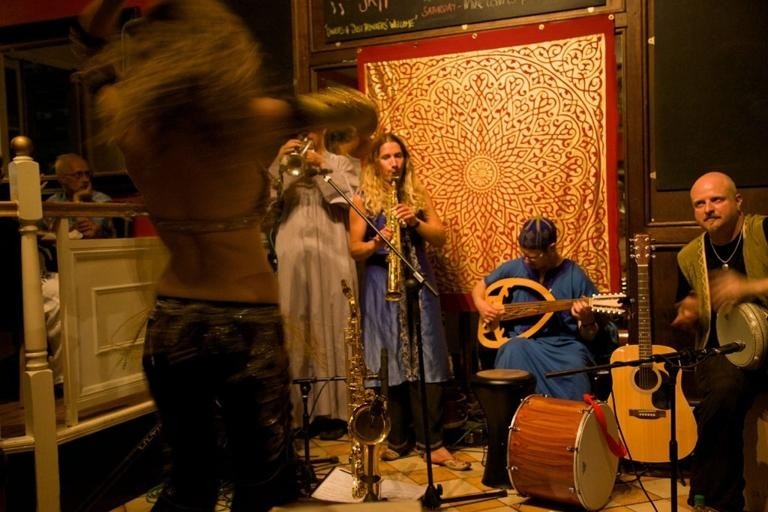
[[340, 278, 392, 502]]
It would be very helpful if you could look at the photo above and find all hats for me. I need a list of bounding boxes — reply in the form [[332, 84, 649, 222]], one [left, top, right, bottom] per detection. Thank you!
[[518, 217, 556, 247]]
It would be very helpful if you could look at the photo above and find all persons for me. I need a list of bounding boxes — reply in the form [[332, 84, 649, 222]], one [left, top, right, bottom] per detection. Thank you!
[[349, 133, 471, 470], [0, 155, 117, 399], [268, 129, 359, 440], [74, 3, 378, 512], [471, 217, 618, 400], [670, 172, 768, 512]]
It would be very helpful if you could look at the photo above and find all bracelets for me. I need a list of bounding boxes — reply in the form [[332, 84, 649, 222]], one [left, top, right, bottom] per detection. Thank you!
[[411, 220, 419, 228]]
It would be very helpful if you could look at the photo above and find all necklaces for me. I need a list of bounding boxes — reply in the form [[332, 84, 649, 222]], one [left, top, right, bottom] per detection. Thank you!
[[710, 228, 742, 271]]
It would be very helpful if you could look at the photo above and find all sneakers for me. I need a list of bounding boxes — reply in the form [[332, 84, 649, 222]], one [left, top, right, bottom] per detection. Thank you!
[[320, 427, 347, 439], [423, 454, 471, 470], [379, 447, 402, 461]]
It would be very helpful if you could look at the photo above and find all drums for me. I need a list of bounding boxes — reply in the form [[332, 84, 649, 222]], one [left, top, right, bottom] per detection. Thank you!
[[505, 392, 620, 510], [715, 302, 768, 367], [473, 370, 537, 493]]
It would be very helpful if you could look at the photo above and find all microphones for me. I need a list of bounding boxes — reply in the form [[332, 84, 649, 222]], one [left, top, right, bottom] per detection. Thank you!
[[380, 345, 389, 398], [700, 340, 746, 357], [306, 168, 334, 177]]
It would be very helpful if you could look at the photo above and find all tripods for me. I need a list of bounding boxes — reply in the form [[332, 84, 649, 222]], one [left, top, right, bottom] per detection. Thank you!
[[302, 409, 340, 482], [408, 292, 510, 512]]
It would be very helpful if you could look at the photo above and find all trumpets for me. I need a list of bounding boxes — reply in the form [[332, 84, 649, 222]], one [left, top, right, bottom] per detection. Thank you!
[[280, 136, 315, 177]]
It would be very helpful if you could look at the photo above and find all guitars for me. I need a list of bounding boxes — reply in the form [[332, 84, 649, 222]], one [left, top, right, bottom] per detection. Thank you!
[[605, 234, 698, 462]]
